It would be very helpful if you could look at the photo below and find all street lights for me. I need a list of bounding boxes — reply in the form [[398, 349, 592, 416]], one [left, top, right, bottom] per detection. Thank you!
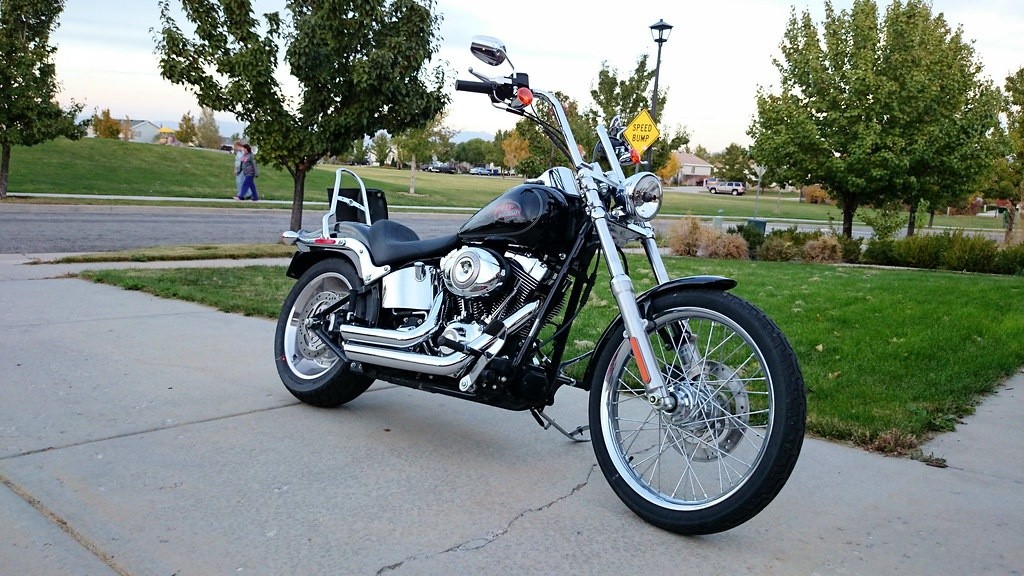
[[644, 18, 674, 175]]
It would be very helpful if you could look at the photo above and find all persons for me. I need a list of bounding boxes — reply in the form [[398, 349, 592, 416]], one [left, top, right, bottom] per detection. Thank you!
[[233, 142, 259, 201]]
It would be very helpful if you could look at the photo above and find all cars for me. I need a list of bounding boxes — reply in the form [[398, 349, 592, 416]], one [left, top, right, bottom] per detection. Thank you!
[[469, 167, 491, 176]]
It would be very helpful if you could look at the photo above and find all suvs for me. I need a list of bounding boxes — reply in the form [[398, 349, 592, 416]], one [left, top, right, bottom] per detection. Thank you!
[[707, 181, 746, 196]]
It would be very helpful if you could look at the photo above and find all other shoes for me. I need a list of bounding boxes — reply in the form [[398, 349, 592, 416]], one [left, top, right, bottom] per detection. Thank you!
[[233, 196, 242, 200], [250, 199, 258, 201]]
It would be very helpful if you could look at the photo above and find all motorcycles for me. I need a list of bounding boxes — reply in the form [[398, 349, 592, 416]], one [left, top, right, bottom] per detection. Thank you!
[[275, 34, 807, 537]]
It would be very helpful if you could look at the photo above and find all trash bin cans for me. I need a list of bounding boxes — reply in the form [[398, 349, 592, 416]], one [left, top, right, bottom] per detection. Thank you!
[[327, 187, 389, 225]]
